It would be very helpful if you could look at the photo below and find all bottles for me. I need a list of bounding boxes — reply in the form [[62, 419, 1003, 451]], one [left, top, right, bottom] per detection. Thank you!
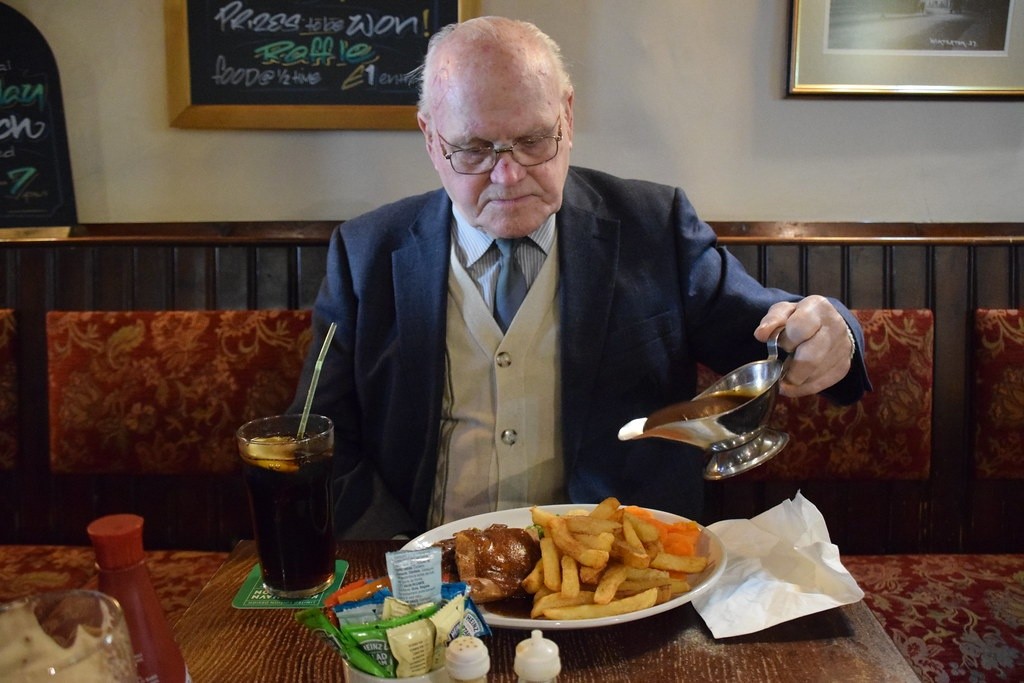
[[445, 627, 491, 683], [511, 629, 563, 683], [86, 512, 191, 683]]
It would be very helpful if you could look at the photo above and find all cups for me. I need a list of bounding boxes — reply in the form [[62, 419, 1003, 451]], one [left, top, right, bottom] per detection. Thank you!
[[237, 412, 337, 601], [0, 588, 136, 683], [342, 656, 450, 682]]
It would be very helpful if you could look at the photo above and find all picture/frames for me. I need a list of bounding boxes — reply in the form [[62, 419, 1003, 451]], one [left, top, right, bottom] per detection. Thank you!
[[164, 1, 481, 133], [790, 1, 1023, 103]]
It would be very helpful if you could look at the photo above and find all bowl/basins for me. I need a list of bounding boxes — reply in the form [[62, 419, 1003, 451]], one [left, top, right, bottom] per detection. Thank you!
[[618, 321, 800, 481]]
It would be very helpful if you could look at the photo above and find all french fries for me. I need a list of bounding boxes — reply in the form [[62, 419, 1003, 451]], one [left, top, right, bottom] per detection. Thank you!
[[522, 498, 710, 620]]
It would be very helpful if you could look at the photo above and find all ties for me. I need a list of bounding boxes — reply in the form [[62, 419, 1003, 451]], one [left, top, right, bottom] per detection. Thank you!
[[493, 238, 526, 335]]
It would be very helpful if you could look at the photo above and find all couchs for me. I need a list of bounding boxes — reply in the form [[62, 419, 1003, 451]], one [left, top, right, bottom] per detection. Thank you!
[[2, 220, 1022, 682]]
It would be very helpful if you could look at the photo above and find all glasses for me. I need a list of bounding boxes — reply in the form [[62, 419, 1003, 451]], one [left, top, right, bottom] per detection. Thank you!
[[422, 96, 565, 176]]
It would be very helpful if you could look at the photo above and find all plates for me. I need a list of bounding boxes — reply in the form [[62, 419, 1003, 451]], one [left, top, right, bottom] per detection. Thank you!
[[390, 501, 728, 632]]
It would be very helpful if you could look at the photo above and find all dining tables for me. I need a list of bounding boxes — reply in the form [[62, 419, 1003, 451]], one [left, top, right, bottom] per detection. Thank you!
[[3, 542, 919, 682]]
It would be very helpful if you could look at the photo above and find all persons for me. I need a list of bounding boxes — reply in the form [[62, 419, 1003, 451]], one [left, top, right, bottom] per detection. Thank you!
[[277, 14, 874, 550]]
